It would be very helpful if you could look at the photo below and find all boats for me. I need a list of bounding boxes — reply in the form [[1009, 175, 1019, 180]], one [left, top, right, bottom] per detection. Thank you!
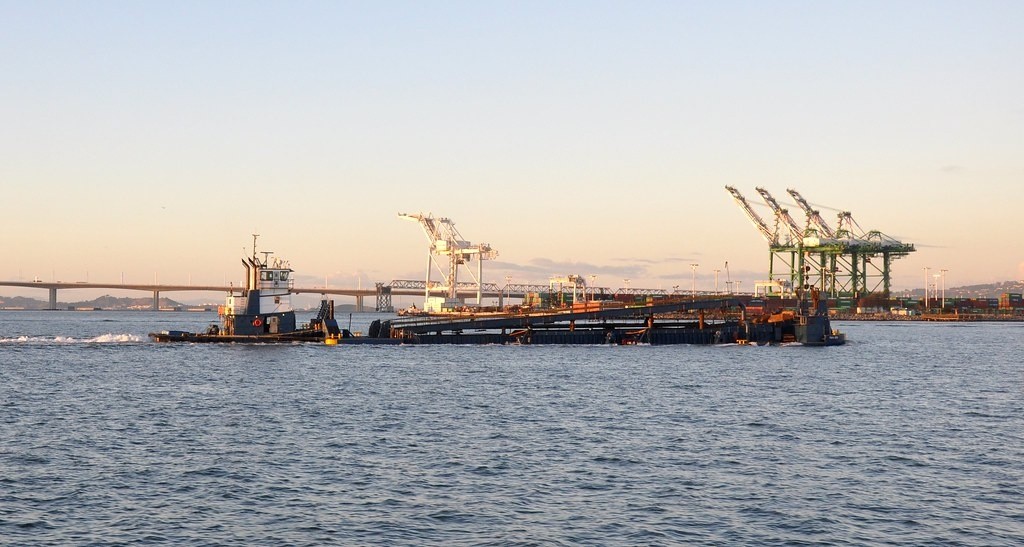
[[143, 233, 357, 342]]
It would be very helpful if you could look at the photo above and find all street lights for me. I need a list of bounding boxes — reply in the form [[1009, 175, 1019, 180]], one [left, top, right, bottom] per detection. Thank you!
[[934, 274, 941, 302], [939, 269, 949, 308], [922, 267, 931, 308]]
